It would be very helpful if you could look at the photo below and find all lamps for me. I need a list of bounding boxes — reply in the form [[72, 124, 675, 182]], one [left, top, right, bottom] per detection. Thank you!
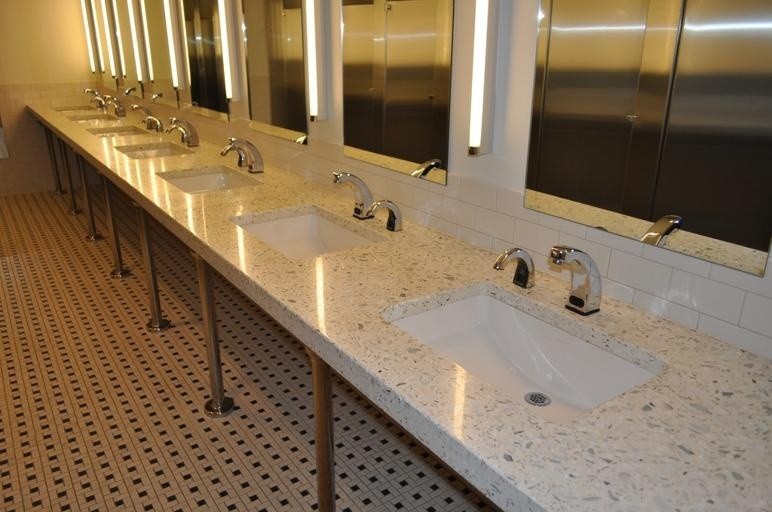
[[305, 1, 318, 123], [80, 0, 96, 76], [163, 2, 179, 92], [217, 1, 233, 103], [179, 0, 192, 89], [112, 1, 128, 80], [90, 1, 107, 75], [139, 1, 156, 85], [125, 1, 144, 86], [101, 0, 118, 80], [464, 0, 498, 158]]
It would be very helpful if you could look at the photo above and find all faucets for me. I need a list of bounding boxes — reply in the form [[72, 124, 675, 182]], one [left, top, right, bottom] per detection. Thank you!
[[492, 247, 536, 290], [410, 159, 442, 179], [364, 199, 404, 233], [332, 171, 375, 220], [294, 135, 307, 144], [547, 245, 602, 315], [52, 72, 264, 197], [641, 213, 683, 245]]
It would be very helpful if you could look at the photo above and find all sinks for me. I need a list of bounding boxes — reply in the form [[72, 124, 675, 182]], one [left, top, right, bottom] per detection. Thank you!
[[380, 280, 669, 424], [228, 204, 390, 262]]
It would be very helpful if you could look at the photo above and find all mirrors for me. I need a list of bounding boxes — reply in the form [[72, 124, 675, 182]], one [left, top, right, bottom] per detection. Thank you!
[[181, 1, 231, 117], [141, 1, 182, 107], [339, 2, 455, 174], [239, 2, 309, 135], [523, 2, 771, 256], [115, 1, 144, 92]]
